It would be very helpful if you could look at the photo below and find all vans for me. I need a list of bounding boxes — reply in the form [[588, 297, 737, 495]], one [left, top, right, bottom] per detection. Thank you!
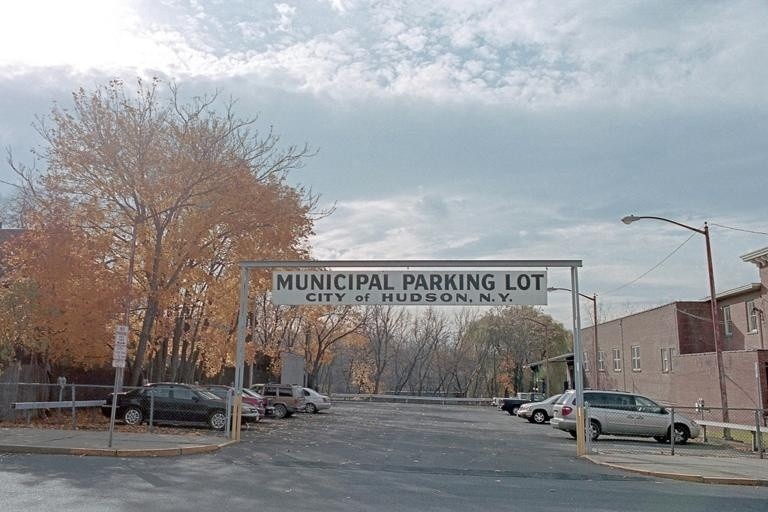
[[549, 387, 701, 445], [248, 384, 306, 418]]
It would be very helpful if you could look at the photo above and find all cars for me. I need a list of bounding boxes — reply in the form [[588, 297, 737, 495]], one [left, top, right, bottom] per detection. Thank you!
[[517, 394, 563, 424], [198, 385, 265, 422], [242, 389, 268, 408], [298, 387, 331, 413], [99, 382, 260, 432]]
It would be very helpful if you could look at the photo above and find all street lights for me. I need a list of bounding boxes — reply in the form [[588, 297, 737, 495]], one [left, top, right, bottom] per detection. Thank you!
[[514, 316, 550, 395], [548, 286, 600, 395], [620, 214, 734, 440]]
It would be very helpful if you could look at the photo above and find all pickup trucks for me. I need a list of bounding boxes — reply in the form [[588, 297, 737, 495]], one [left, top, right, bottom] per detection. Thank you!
[[491, 392, 545, 415]]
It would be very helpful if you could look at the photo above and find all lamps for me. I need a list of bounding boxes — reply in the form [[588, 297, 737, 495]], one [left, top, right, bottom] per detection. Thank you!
[[749, 306, 764, 322]]
